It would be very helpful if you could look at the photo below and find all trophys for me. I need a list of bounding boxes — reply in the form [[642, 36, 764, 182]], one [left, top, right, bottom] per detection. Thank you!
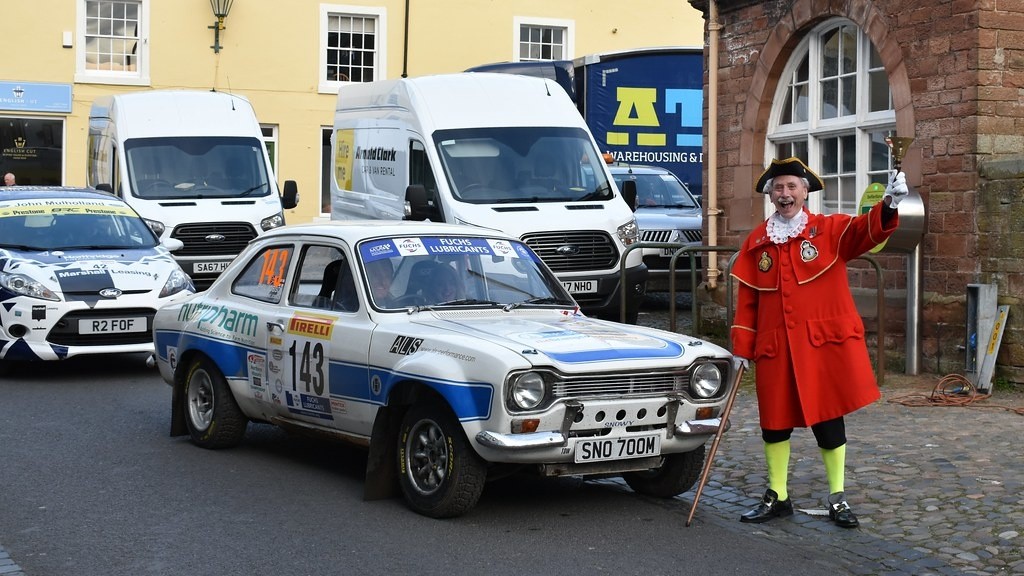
[[883, 135, 917, 195]]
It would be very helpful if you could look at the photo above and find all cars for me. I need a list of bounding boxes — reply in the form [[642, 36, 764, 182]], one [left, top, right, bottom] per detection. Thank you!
[[146, 213, 739, 520], [1, 184, 198, 381]]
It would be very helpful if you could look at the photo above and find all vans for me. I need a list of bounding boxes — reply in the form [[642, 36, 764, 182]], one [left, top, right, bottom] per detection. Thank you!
[[86, 87, 300, 291], [327, 68, 652, 326], [577, 154, 704, 280]]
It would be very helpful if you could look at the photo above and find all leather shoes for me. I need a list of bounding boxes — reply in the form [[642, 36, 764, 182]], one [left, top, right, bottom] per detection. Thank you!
[[828, 491, 859, 528], [740, 488, 793, 523]]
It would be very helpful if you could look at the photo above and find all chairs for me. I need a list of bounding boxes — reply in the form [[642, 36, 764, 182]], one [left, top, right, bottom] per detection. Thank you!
[[310, 259, 344, 310], [404, 258, 465, 305]]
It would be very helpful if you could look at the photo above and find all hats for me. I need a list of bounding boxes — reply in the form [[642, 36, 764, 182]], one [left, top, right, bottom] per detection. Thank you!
[[756, 158, 824, 193]]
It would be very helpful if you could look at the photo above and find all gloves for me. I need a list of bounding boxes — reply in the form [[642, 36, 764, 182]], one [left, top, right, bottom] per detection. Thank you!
[[882, 169, 909, 210], [732, 355, 750, 374]]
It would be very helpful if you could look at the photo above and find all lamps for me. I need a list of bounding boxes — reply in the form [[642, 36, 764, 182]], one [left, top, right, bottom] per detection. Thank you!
[[207, 0, 235, 56]]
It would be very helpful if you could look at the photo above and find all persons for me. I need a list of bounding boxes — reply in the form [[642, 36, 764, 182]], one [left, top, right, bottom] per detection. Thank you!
[[338, 255, 476, 312], [729, 157, 908, 530], [4, 173, 17, 187]]
